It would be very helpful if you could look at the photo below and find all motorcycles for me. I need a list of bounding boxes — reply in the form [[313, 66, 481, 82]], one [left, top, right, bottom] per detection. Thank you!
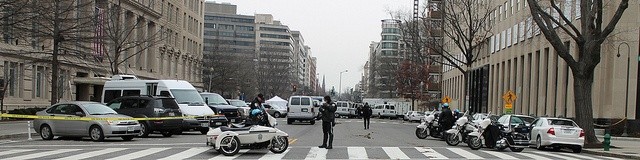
[[466, 111, 531, 152], [415, 106, 447, 139], [445, 110, 489, 146], [205, 103, 289, 156]]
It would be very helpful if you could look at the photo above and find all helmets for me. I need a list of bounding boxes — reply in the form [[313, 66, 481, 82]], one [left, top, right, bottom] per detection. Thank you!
[[454, 109, 459, 112], [442, 103, 449, 107], [252, 109, 261, 116]]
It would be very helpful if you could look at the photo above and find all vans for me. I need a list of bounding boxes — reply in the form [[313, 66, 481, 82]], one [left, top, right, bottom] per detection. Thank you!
[[370, 104, 397, 120], [101, 73, 215, 134], [287, 96, 315, 125], [335, 101, 353, 119]]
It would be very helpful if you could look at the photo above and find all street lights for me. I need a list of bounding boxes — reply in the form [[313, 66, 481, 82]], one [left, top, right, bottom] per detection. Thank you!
[[339, 70, 348, 101], [617, 42, 630, 137]]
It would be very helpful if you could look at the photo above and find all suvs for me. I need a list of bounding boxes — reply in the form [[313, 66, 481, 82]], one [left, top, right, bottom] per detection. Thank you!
[[199, 92, 241, 124], [226, 99, 251, 122], [247, 102, 288, 118], [105, 95, 183, 137]]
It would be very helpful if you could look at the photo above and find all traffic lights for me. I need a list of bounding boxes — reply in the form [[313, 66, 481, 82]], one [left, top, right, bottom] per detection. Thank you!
[[293, 85, 296, 91]]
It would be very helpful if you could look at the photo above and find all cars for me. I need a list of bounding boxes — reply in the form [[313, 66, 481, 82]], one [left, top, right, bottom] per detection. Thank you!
[[480, 114, 500, 129], [32, 100, 142, 142], [470, 114, 487, 128], [529, 117, 585, 153], [420, 109, 443, 124], [403, 111, 413, 121], [408, 111, 425, 123], [495, 114, 536, 144]]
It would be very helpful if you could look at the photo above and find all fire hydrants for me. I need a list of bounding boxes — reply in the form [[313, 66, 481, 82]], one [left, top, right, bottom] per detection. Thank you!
[[602, 133, 611, 151]]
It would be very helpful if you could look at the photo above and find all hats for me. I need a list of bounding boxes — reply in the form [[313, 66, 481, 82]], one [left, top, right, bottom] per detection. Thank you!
[[366, 102, 368, 104], [324, 96, 330, 101], [258, 93, 265, 100]]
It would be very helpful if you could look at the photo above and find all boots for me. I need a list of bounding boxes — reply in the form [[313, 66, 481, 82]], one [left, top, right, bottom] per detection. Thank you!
[[326, 134, 333, 149], [319, 133, 328, 147]]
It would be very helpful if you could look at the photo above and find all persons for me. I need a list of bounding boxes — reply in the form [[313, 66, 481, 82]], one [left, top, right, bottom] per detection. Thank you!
[[432, 108, 439, 119], [318, 96, 337, 149], [439, 102, 452, 141], [358, 107, 362, 119], [250, 93, 265, 109], [453, 109, 460, 120], [363, 103, 372, 129], [245, 109, 269, 127]]
